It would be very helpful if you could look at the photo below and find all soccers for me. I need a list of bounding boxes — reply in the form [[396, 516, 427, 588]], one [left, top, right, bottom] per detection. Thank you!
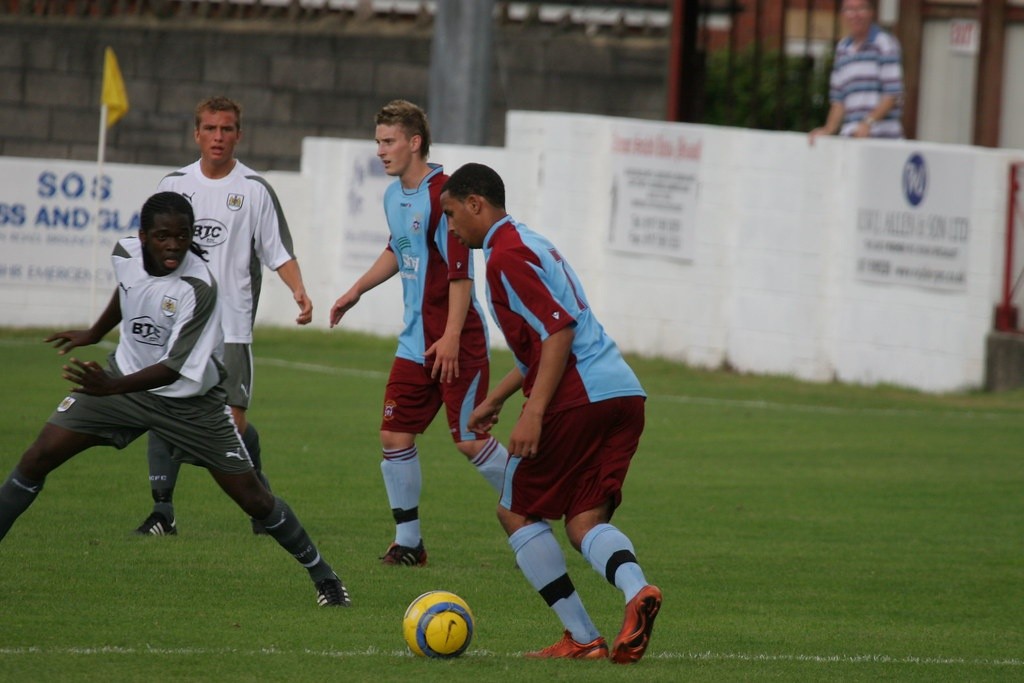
[[402, 589, 475, 659]]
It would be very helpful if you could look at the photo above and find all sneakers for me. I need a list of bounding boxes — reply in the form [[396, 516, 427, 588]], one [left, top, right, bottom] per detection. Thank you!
[[135, 511, 178, 537], [314, 571, 351, 608], [250, 475, 270, 536], [523, 629, 609, 660], [609, 583, 662, 664], [377, 538, 428, 567]]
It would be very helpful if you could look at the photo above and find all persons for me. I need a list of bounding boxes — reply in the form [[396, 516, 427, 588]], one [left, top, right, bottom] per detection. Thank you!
[[808, 0, 903, 142], [0, 192, 350, 611], [439, 163, 661, 660], [329, 99, 510, 567], [138, 96, 313, 538]]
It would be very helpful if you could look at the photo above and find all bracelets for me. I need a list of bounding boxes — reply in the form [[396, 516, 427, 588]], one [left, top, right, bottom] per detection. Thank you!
[[865, 117, 875, 126]]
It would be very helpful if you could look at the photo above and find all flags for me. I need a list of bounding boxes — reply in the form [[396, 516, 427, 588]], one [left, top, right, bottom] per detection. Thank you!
[[101, 49, 130, 128]]
